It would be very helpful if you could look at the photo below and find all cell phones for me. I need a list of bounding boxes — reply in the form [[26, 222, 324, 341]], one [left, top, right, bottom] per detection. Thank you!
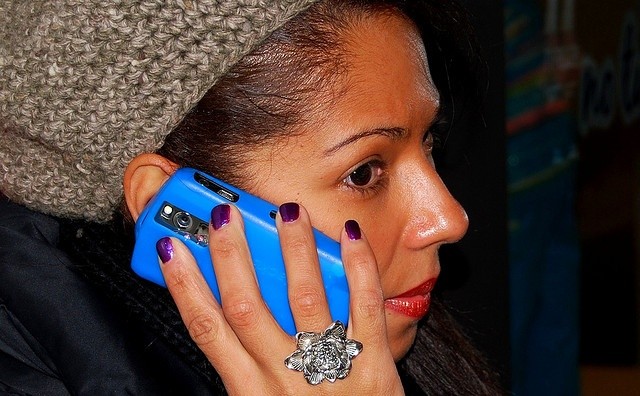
[[129, 166, 351, 335]]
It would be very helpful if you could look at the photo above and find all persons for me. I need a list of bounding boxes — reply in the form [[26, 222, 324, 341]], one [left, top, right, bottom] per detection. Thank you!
[[0, 2, 471, 396]]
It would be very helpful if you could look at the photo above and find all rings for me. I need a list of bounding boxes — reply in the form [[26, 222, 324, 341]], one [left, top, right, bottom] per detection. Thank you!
[[283, 319, 363, 386]]
